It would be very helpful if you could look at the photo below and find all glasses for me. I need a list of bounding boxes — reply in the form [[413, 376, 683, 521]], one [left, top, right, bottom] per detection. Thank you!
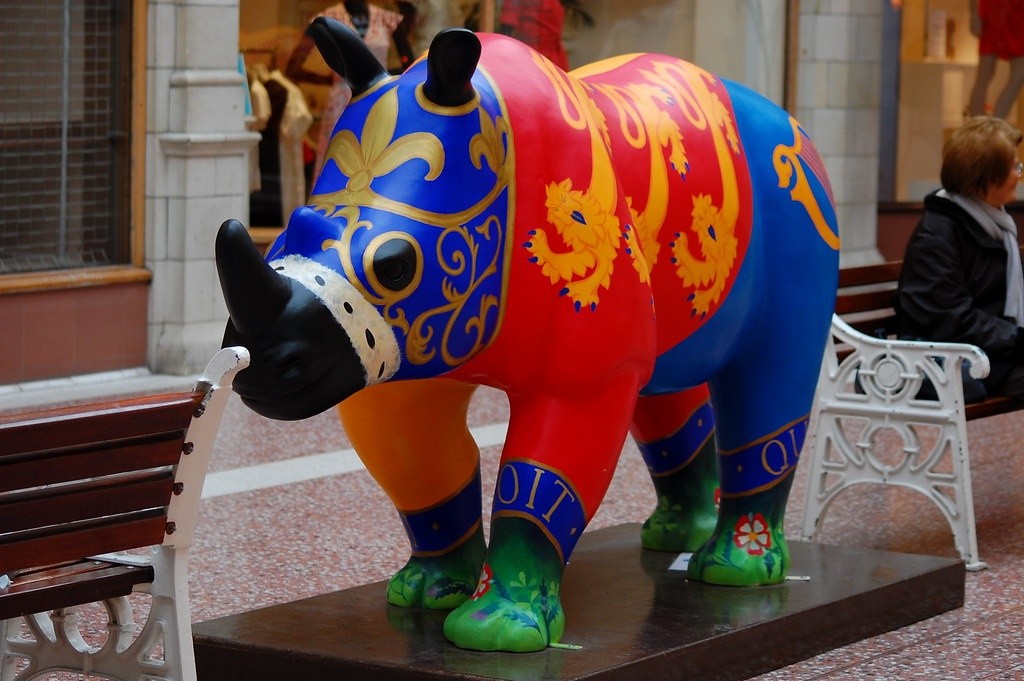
[[1011, 162, 1023, 173]]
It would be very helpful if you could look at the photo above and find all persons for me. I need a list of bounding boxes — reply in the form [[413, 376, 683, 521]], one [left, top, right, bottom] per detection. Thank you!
[[895, 114, 1024, 396]]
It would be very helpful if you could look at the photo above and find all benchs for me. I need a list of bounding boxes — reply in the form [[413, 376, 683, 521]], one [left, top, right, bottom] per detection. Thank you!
[[803, 262, 1024, 572], [0, 346, 250, 681]]
